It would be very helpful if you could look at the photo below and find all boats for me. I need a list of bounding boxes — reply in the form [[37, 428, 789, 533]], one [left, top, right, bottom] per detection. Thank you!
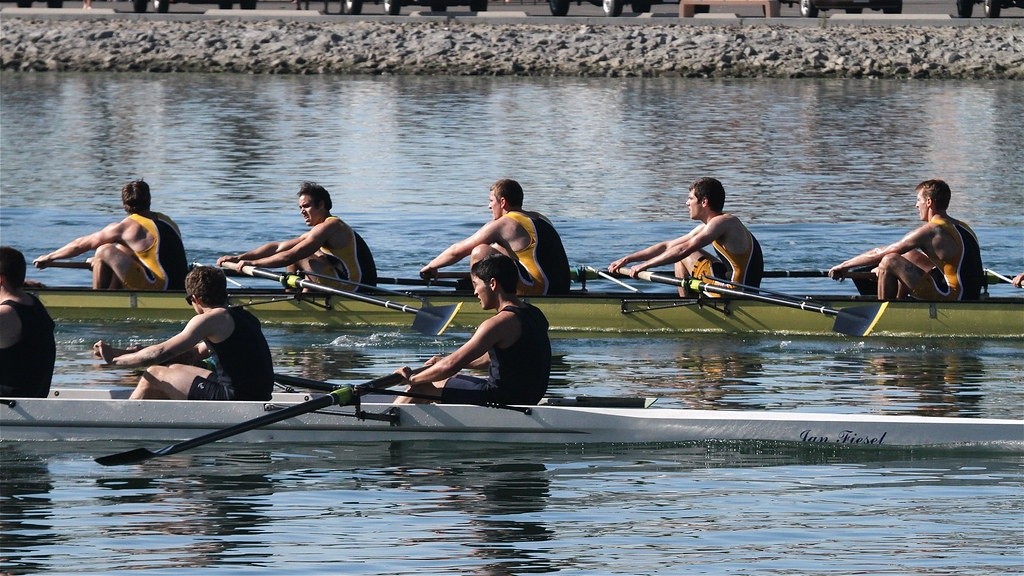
[[0, 389, 1024, 444], [31, 281, 1024, 336]]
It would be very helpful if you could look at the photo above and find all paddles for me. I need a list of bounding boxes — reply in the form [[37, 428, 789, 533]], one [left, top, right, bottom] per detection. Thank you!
[[417, 260, 1024, 338], [91, 346, 659, 468], [32, 261, 465, 336]]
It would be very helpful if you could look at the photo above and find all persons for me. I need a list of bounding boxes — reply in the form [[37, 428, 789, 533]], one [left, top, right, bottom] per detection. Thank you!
[[33, 180, 189, 291], [83, 0, 92, 9], [392, 254, 552, 406], [1013, 273, 1024, 288], [297, 0, 309, 10], [419, 179, 571, 296], [216, 181, 377, 293], [828, 180, 983, 302], [608, 177, 763, 297], [0, 247, 57, 399], [94, 266, 275, 402]]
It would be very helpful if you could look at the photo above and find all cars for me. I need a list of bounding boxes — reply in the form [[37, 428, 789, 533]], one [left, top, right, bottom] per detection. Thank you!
[[16, 0, 63, 8], [545, 0, 711, 17], [346, 0, 487, 16], [956, 0, 1024, 18], [132, 0, 257, 13], [761, 0, 903, 17]]
